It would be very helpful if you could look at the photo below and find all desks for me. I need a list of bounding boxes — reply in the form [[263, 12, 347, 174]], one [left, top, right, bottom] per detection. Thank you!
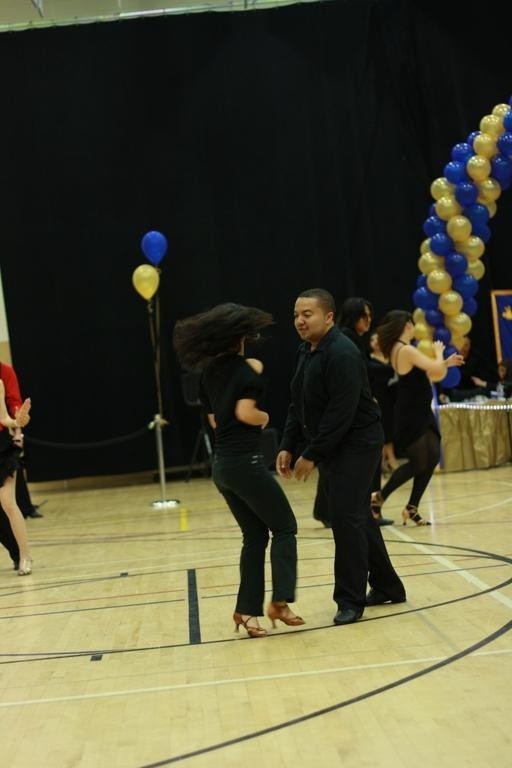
[[394, 399, 512, 474]]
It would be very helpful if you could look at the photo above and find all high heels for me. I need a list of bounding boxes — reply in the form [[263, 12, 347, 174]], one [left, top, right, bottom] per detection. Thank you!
[[378, 461, 401, 479], [401, 504, 432, 527], [267, 600, 307, 630], [232, 612, 268, 638]]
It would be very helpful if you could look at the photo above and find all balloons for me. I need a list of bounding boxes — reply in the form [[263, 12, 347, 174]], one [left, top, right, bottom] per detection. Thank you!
[[141, 231, 167, 265], [132, 264, 160, 300], [411, 102, 511, 390]]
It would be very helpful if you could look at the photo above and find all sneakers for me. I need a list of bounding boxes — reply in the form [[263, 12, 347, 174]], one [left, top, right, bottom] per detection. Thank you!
[[12, 553, 33, 576], [23, 509, 44, 520], [368, 490, 383, 522]]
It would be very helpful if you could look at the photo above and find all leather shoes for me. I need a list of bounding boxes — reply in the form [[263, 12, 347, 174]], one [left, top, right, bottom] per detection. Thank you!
[[360, 588, 408, 608], [333, 602, 364, 625]]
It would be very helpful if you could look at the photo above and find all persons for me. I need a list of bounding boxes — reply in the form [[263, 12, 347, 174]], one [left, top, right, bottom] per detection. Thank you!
[[274, 287, 406, 624], [173, 303, 304, 639], [0, 378, 32, 575], [0, 357, 44, 571]]
